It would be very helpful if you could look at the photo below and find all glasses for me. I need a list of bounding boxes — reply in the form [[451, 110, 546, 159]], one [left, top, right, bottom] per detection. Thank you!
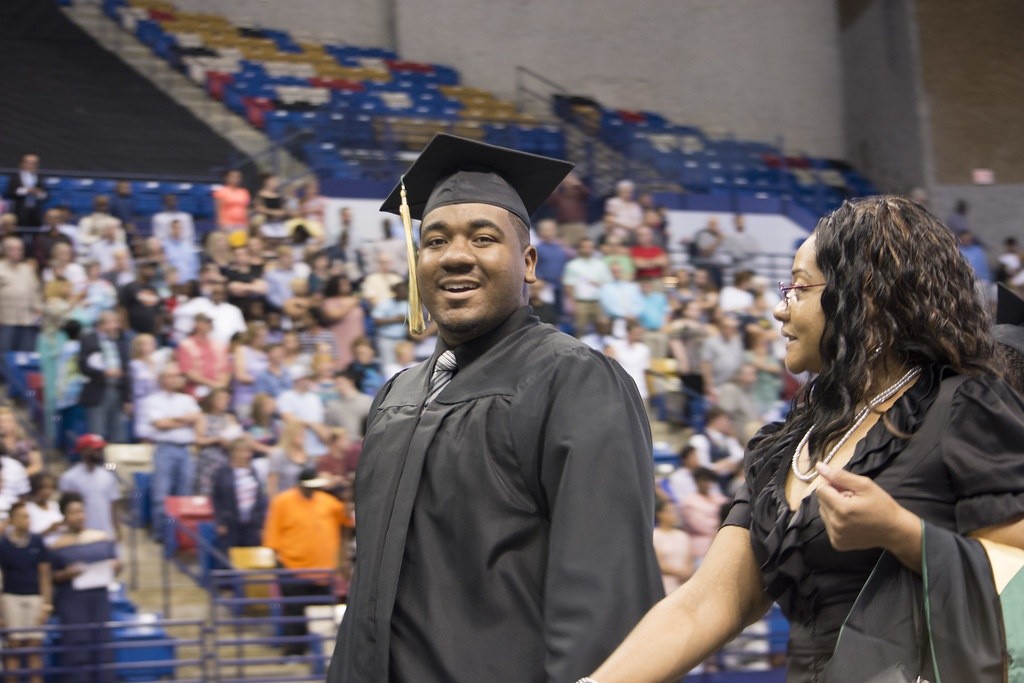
[[777, 282, 827, 305]]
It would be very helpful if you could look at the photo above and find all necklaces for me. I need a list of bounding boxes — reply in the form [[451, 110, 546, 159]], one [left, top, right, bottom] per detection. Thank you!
[[791, 365, 923, 480]]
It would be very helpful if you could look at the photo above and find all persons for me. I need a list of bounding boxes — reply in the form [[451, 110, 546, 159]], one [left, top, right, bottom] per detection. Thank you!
[[0, 404, 126, 683], [334, 175, 812, 678], [324, 133, 666, 683], [0, 154, 357, 657], [911, 191, 1023, 325], [577, 195, 1024, 683]]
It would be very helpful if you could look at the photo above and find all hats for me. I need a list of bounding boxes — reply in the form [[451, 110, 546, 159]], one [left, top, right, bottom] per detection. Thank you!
[[72, 433, 108, 454], [296, 469, 329, 489], [58, 320, 81, 332], [379, 132, 575, 336]]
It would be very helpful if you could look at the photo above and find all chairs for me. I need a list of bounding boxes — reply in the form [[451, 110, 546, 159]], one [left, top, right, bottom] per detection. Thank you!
[[1, 0, 885, 226]]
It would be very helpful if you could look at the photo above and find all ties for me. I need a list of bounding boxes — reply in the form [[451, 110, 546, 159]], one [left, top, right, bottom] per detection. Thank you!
[[419, 349, 458, 418]]
